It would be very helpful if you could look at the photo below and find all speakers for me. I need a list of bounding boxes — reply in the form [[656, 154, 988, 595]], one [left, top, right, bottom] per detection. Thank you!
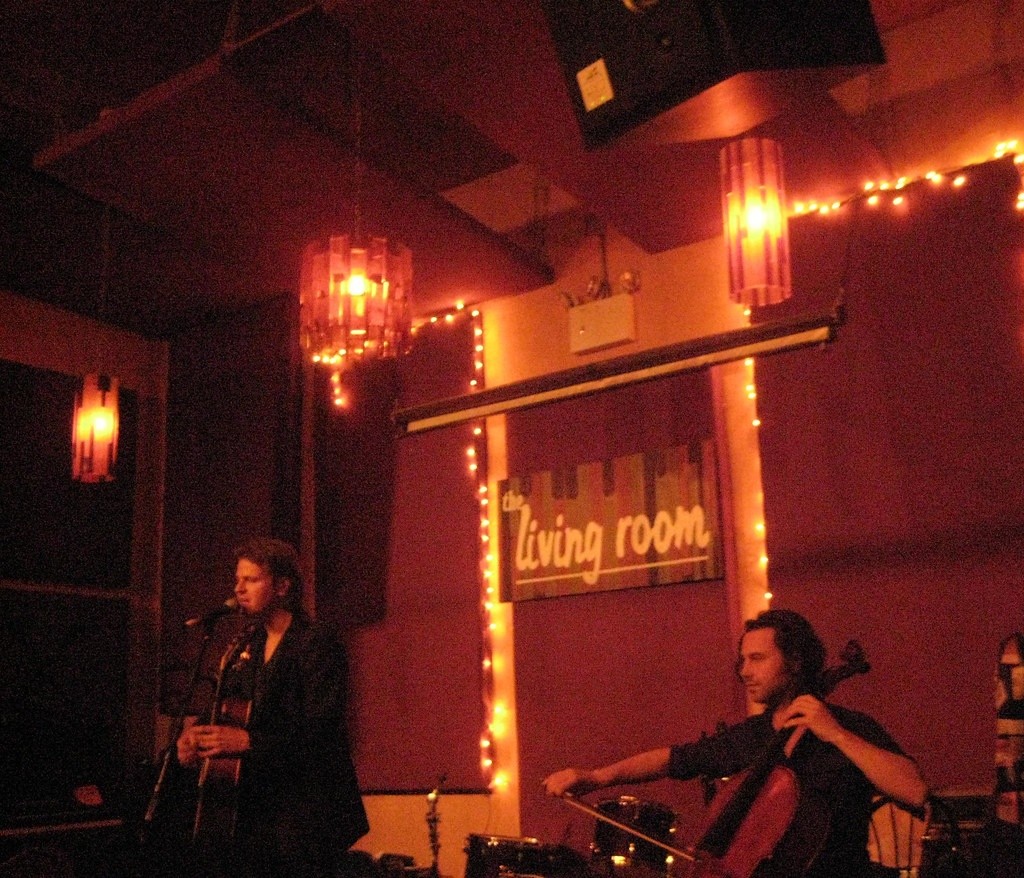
[[544, 0, 888, 153]]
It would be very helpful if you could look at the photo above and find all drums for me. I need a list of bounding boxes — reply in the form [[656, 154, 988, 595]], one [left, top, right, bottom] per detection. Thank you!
[[588, 797, 675, 875], [463, 831, 583, 878]]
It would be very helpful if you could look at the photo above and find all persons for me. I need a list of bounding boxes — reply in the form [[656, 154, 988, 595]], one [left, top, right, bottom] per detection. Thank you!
[[542, 609, 927, 878], [173, 538, 371, 870]]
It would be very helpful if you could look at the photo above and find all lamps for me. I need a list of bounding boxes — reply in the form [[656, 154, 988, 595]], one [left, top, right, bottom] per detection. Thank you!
[[721, 131, 792, 307], [298, 81, 413, 365], [71, 208, 119, 483], [559, 270, 641, 355]]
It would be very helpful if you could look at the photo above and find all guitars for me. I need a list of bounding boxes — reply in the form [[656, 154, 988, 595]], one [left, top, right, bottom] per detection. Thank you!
[[190, 620, 258, 847]]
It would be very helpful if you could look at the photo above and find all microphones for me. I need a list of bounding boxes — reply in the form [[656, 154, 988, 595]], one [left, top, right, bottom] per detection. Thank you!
[[183, 597, 242, 632]]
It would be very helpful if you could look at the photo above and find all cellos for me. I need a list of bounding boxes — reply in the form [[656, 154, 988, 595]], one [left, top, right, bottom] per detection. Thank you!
[[668, 641, 870, 878]]
